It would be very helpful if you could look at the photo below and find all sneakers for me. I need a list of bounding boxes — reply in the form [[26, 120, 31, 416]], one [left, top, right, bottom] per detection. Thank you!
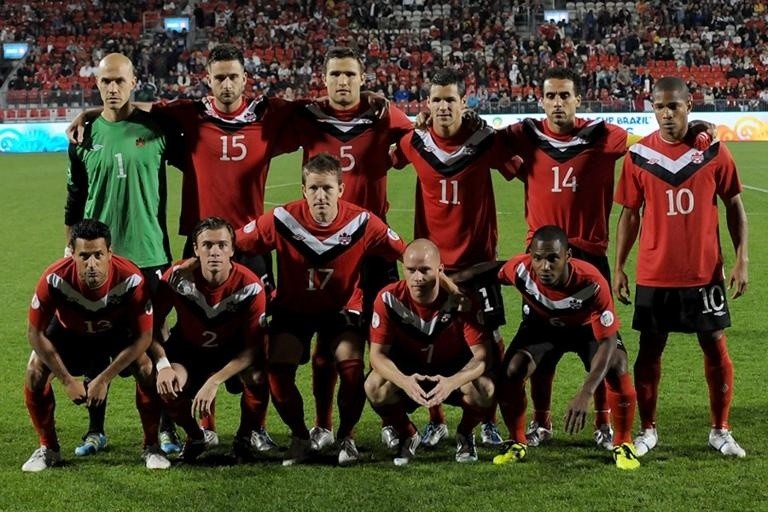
[[593, 423, 613, 451], [232, 426, 360, 466], [22, 444, 60, 472], [632, 427, 658, 458], [421, 423, 449, 447], [525, 421, 552, 447], [380, 424, 400, 449], [492, 439, 527, 465], [708, 427, 746, 458], [141, 427, 219, 469], [455, 431, 478, 462], [480, 423, 504, 448], [393, 432, 421, 466], [613, 442, 640, 470], [75, 432, 108, 457]]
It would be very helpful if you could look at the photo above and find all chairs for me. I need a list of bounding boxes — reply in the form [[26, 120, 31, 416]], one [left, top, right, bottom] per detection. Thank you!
[[0, 0, 768, 123]]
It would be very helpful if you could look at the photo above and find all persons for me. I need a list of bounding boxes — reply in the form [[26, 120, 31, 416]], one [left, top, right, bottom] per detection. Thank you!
[[447, 226, 641, 471], [63, 53, 183, 458], [65, 42, 390, 451], [415, 65, 717, 451], [362, 238, 498, 468], [388, 67, 524, 448], [290, 47, 416, 451], [0, 0, 768, 116], [173, 152, 474, 468], [21, 219, 172, 471], [612, 76, 749, 458], [147, 215, 279, 466]]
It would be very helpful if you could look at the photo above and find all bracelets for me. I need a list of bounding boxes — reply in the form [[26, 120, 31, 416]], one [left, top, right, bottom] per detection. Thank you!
[[155, 357, 172, 372]]
